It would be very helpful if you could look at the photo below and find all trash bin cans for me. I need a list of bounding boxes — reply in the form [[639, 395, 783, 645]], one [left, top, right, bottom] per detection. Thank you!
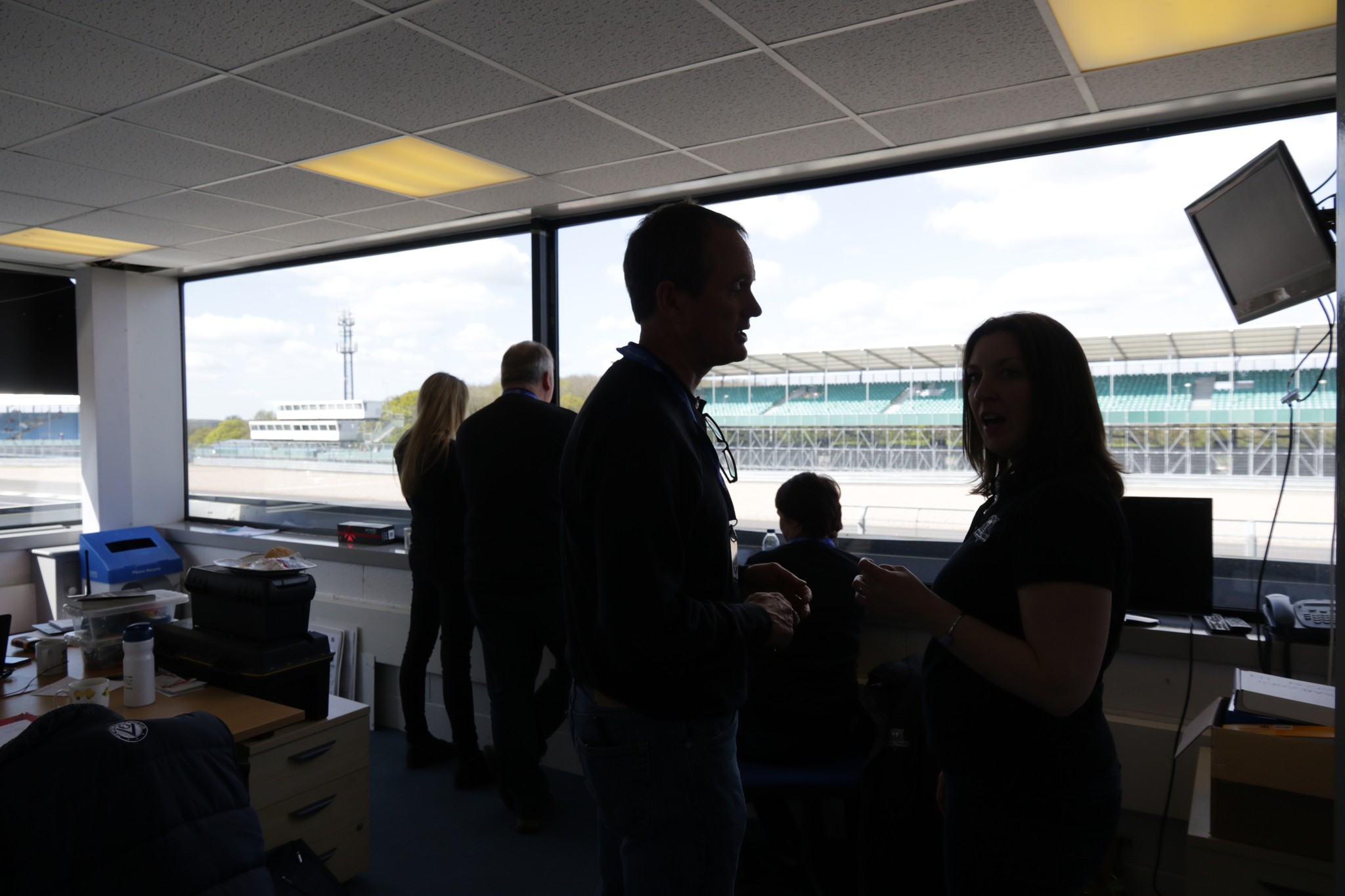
[[78, 525, 184, 593]]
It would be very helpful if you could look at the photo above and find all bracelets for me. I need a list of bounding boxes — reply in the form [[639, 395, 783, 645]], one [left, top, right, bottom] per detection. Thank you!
[[938, 607, 963, 646]]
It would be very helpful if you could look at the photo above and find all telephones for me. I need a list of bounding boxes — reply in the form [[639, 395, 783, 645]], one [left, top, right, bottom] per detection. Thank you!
[[1264, 592, 1338, 637]]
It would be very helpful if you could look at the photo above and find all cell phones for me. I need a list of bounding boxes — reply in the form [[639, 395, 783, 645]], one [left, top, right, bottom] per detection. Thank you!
[[5, 656, 30, 666]]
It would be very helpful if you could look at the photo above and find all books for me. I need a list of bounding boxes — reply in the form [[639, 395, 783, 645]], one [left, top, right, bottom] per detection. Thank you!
[[1232, 668, 1336, 728]]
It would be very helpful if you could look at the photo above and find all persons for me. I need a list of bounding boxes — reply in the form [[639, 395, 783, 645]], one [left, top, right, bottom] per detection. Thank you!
[[392, 373, 497, 788], [566, 200, 808, 896], [733, 313, 1122, 896], [457, 341, 577, 794]]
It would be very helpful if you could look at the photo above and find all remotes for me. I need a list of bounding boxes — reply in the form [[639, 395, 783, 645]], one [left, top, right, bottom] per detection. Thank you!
[[1222, 616, 1252, 632], [1203, 613, 1231, 634]]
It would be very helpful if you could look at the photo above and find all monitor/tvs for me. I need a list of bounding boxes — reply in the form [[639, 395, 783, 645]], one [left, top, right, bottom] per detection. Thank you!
[[1121, 494, 1214, 627], [1184, 140, 1336, 324]]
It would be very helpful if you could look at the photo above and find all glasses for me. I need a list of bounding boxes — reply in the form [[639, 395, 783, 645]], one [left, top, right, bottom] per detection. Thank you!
[[702, 413, 738, 483]]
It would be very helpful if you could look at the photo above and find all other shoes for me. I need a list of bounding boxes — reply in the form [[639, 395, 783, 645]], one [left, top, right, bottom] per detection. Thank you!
[[456, 754, 495, 791], [517, 795, 552, 831], [482, 746, 514, 812], [405, 732, 456, 769]]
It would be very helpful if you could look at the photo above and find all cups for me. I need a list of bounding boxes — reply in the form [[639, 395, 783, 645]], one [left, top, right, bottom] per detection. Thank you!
[[404, 527, 412, 551], [55, 677, 110, 708], [35, 639, 68, 677]]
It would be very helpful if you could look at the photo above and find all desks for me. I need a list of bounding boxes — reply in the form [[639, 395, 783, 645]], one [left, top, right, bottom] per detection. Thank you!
[[0, 630, 305, 747]]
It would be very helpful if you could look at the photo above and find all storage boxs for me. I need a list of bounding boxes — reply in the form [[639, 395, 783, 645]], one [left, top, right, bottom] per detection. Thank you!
[[161, 619, 331, 742], [62, 589, 189, 639], [1175, 668, 1336, 861], [64, 629, 123, 670], [183, 564, 316, 643]]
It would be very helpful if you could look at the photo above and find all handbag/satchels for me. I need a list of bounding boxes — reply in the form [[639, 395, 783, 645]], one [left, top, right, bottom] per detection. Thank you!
[[264, 839, 347, 896]]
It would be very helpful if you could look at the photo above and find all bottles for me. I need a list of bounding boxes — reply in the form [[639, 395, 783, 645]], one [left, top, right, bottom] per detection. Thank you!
[[122, 621, 156, 707], [762, 529, 779, 550]]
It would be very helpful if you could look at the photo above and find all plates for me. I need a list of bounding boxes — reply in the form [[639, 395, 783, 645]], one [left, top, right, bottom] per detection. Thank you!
[[214, 557, 317, 574]]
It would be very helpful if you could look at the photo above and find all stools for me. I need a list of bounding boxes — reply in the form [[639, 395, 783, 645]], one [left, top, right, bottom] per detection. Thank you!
[[739, 763, 867, 896]]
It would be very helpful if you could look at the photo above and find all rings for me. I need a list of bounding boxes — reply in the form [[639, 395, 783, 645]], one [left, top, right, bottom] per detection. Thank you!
[[858, 584, 868, 594]]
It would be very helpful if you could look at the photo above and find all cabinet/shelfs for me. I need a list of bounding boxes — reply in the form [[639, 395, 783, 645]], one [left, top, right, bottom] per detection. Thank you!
[[247, 716, 371, 883], [1185, 847, 1335, 896]]
